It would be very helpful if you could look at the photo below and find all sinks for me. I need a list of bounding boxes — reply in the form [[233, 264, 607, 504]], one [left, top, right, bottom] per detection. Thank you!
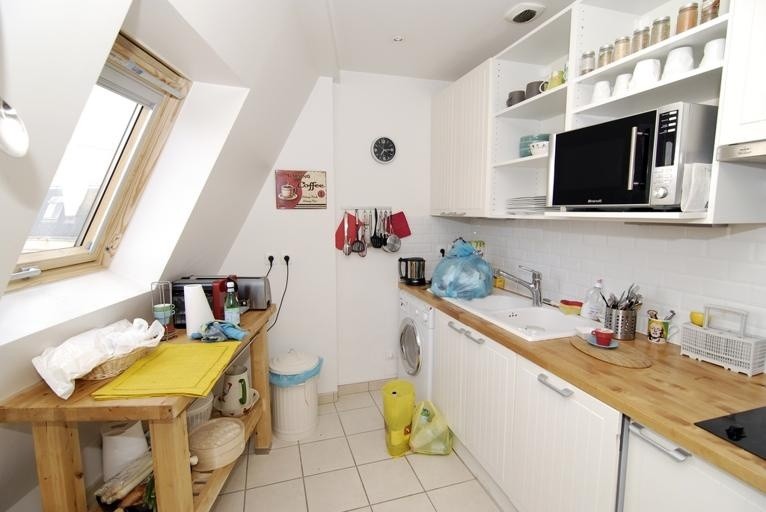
[[485, 308, 605, 343], [426, 285, 534, 312]]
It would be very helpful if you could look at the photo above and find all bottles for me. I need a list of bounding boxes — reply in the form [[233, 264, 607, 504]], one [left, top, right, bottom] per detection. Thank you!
[[224, 282, 241, 327], [578, 0, 726, 78]]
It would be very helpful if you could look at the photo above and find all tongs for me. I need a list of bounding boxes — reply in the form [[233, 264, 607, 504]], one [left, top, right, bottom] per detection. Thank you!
[[343, 213, 352, 256]]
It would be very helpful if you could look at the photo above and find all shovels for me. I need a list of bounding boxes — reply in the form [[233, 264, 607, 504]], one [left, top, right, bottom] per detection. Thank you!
[[371, 208, 382, 248], [350, 209, 365, 252]]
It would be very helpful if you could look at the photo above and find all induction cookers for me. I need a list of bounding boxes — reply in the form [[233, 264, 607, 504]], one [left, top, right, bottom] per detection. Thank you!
[[695, 404, 766, 460]]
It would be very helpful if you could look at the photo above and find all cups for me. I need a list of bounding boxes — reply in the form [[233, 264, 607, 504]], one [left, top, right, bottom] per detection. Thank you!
[[501, 64, 566, 108], [280, 184, 296, 198], [590, 329, 615, 345], [152, 302, 175, 335], [647, 317, 680, 344]]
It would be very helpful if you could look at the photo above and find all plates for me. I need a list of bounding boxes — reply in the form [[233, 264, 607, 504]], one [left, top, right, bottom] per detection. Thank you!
[[505, 196, 559, 215], [277, 193, 298, 200], [588, 338, 620, 349]]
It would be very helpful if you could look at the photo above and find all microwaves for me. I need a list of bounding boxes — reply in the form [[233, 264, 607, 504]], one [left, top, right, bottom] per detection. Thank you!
[[546, 102, 717, 209]]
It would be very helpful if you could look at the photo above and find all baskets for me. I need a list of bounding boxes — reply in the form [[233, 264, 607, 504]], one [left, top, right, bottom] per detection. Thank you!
[[81, 348, 147, 380]]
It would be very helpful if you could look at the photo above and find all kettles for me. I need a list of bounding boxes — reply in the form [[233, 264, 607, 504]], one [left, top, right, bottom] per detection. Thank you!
[[221, 366, 250, 411], [398, 257, 427, 286]]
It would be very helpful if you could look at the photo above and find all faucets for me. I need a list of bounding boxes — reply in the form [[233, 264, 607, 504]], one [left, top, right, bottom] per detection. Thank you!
[[493, 264, 543, 308]]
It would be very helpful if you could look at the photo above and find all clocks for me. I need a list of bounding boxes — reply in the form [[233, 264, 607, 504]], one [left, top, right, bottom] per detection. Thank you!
[[371, 135, 397, 164]]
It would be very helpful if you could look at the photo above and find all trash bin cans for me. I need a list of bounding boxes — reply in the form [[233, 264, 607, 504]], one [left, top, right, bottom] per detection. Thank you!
[[268, 348, 320, 442]]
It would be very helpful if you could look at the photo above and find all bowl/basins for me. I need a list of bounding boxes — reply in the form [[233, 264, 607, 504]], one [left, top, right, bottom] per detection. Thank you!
[[96, 493, 152, 511], [518, 132, 550, 158], [213, 387, 260, 414]]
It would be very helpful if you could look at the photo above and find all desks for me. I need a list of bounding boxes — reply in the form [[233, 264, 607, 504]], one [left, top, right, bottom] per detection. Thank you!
[[0, 303, 278, 512]]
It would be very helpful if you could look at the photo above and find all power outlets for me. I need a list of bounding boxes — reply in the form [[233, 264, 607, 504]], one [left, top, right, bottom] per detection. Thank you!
[[281, 252, 292, 265], [265, 251, 277, 265]]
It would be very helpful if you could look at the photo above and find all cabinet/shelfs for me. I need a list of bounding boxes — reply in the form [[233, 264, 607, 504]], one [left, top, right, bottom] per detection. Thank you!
[[487, 4, 571, 220], [622, 417, 766, 512], [506, 354, 621, 511], [430, 57, 487, 218], [430, 309, 515, 509]]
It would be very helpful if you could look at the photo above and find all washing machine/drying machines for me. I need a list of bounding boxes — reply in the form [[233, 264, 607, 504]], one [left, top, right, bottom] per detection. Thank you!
[[398, 288, 435, 405]]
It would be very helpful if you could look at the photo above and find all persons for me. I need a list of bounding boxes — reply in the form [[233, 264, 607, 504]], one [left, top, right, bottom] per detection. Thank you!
[[648, 319, 666, 344]]
